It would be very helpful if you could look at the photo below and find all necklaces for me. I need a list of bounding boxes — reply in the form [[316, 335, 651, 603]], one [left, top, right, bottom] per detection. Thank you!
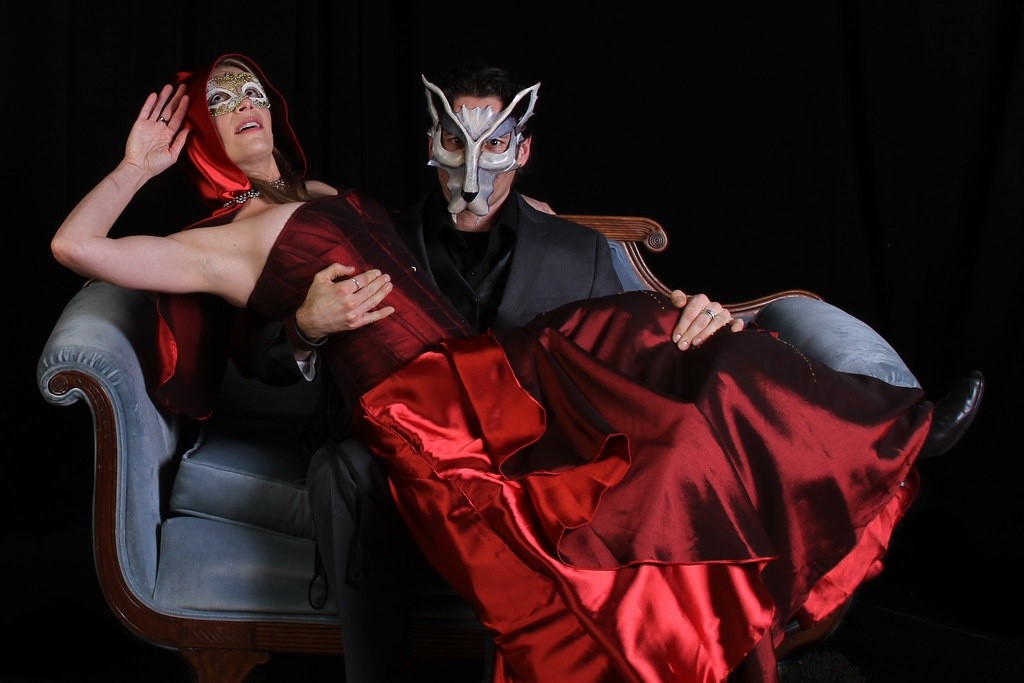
[[217, 173, 290, 213]]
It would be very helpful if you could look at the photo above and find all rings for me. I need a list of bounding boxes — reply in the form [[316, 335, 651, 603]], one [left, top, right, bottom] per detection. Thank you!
[[158, 115, 168, 124], [349, 275, 361, 292], [701, 307, 715, 322]]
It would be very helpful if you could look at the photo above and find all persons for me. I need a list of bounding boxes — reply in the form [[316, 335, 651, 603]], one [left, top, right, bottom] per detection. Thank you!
[[50, 47, 1000, 683], [258, 56, 744, 682]]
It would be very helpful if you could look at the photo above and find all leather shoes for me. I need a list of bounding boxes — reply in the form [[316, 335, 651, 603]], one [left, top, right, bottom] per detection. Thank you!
[[919, 368, 986, 459]]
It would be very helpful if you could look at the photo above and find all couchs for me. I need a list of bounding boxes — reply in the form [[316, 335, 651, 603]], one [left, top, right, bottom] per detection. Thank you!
[[36, 213, 923, 683]]
